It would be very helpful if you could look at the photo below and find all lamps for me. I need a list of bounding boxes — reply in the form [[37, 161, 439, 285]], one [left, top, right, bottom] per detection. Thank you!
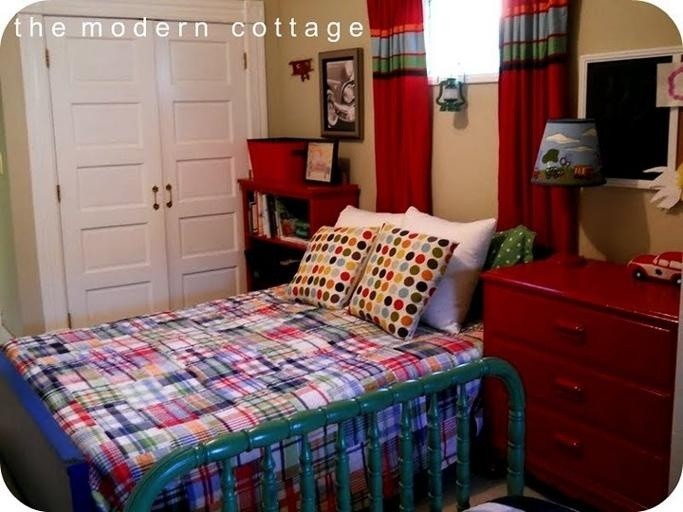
[[436, 78, 466, 112], [529, 120, 608, 266]]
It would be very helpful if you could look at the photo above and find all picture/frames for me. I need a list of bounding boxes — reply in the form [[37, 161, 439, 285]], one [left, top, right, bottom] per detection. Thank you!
[[305, 138, 339, 184], [577, 45, 682, 190], [318, 48, 364, 143]]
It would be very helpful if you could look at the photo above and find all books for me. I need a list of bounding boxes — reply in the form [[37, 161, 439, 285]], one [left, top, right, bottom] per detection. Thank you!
[[246, 190, 311, 247]]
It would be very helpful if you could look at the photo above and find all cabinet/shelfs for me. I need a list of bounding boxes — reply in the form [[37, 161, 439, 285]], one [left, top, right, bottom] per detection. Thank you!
[[1, 1, 268, 335], [481, 255, 683, 512], [237, 180, 359, 300]]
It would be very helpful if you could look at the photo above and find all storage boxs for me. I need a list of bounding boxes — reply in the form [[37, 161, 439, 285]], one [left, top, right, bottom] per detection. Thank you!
[[247, 137, 308, 184]]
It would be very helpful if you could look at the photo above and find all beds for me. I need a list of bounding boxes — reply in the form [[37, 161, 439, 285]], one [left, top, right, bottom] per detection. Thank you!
[[123, 357, 581, 512], [1, 283, 485, 512]]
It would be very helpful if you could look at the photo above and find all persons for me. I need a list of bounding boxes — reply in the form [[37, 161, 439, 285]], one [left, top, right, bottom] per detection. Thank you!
[[624, 250, 682, 287]]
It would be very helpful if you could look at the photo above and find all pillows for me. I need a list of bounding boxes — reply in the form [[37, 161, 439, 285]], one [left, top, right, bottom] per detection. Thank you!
[[284, 202, 536, 341]]
